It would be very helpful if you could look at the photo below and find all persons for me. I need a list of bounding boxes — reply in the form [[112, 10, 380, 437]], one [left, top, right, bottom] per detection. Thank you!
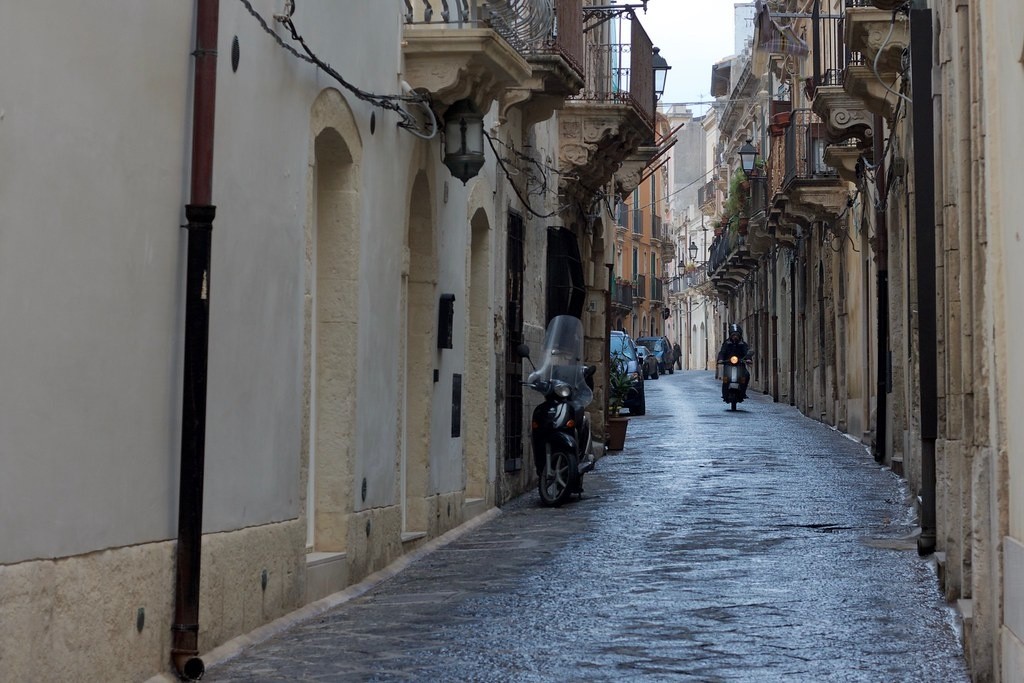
[[715, 323, 756, 397]]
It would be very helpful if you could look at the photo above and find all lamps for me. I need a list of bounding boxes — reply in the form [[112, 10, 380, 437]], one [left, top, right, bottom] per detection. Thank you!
[[737, 136, 768, 184], [688, 242, 698, 260], [440, 96, 486, 186], [677, 261, 685, 277], [651, 46, 672, 100]]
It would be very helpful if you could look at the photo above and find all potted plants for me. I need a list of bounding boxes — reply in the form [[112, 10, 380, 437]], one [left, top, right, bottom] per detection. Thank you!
[[713, 156, 765, 238], [797, 73, 814, 101]]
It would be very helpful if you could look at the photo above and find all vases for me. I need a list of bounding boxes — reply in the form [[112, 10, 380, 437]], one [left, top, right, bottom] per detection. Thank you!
[[774, 112, 790, 127], [770, 123, 784, 135]]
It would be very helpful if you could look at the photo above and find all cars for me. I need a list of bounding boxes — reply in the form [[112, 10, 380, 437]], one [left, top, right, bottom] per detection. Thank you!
[[634, 345, 659, 381], [609, 329, 646, 418]]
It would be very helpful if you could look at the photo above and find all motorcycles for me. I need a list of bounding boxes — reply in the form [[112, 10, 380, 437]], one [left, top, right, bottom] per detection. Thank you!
[[517, 315, 597, 507], [717, 354, 752, 411]]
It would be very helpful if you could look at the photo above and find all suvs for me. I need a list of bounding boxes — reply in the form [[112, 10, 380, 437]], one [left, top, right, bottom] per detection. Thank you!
[[635, 335, 674, 375]]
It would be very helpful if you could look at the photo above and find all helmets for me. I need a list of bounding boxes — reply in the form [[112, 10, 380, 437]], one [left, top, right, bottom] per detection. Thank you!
[[729, 323, 743, 335]]
